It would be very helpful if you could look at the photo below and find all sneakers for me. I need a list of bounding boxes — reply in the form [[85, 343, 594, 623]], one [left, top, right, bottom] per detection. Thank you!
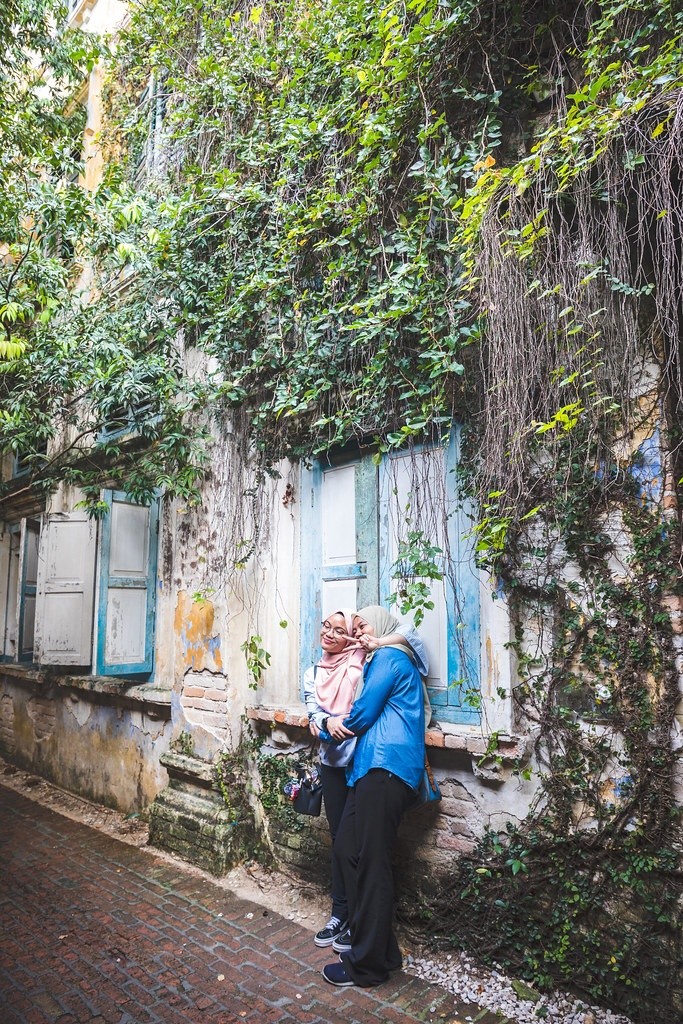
[[330, 927, 351, 954], [313, 915, 351, 948], [320, 962, 389, 986], [339, 950, 404, 970]]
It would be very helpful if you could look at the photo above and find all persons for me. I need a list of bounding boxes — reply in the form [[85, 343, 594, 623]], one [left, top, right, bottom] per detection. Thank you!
[[312, 606, 426, 987], [303, 609, 430, 952]]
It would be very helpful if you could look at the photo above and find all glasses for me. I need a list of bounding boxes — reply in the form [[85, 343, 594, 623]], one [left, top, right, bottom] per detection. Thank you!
[[321, 621, 347, 638]]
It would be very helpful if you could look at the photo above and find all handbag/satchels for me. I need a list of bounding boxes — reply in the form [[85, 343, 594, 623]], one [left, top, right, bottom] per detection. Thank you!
[[284, 738, 323, 817], [418, 753, 442, 804]]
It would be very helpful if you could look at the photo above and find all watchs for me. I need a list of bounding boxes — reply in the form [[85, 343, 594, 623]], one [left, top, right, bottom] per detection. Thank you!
[[322, 717, 329, 732]]
